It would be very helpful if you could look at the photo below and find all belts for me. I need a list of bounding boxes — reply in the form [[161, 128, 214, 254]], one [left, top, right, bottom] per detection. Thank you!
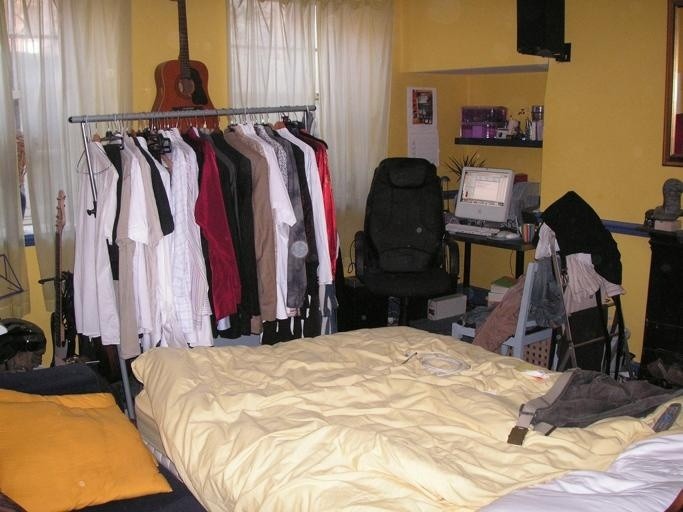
[[508, 367, 581, 445]]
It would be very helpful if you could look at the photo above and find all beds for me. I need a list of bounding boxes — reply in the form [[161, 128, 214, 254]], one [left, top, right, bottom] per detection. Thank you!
[[127, 323, 683, 510]]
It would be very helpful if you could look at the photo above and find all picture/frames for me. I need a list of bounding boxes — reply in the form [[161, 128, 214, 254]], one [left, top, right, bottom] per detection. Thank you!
[[660, 0, 683, 168]]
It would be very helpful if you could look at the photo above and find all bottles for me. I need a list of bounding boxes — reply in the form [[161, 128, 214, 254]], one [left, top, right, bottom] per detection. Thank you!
[[531, 105, 544, 141]]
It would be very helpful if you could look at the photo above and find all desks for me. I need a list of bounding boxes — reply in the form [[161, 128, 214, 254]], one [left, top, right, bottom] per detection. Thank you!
[[634, 224, 683, 389], [444, 219, 539, 305]]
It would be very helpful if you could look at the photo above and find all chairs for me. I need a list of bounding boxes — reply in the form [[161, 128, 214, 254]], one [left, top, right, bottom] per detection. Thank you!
[[0, 359, 190, 511], [355, 155, 460, 328], [450, 257, 555, 368]]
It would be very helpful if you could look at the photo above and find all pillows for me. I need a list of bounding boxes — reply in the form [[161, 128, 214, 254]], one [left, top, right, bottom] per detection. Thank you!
[[0, 384, 173, 512]]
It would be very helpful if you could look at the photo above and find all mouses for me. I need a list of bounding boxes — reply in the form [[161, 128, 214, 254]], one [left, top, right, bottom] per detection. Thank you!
[[506, 233, 520, 239]]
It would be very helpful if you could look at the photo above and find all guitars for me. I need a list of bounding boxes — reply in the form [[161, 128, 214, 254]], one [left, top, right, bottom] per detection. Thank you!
[[50, 189, 75, 366], [149, 0, 219, 137]]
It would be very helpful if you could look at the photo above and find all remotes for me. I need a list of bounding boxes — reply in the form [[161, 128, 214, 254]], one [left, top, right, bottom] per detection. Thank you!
[[651, 403, 681, 433]]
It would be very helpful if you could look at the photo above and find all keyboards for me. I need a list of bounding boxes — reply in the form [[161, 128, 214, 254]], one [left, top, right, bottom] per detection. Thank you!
[[445, 223, 500, 236]]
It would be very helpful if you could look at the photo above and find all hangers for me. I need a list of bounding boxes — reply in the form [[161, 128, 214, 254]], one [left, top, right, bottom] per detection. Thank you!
[[75, 104, 313, 175]]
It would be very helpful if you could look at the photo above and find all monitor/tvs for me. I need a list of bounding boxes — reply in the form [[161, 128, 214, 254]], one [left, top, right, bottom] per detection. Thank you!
[[454, 167, 515, 228]]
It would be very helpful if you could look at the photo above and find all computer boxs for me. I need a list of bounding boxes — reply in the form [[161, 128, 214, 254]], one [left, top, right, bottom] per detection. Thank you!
[[426, 292, 468, 321]]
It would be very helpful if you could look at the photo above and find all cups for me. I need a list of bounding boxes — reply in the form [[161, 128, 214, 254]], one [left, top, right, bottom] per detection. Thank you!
[[519, 223, 535, 243]]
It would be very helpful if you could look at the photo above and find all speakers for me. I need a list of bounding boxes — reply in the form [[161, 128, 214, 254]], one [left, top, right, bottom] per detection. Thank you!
[[516, 0, 564, 58]]
